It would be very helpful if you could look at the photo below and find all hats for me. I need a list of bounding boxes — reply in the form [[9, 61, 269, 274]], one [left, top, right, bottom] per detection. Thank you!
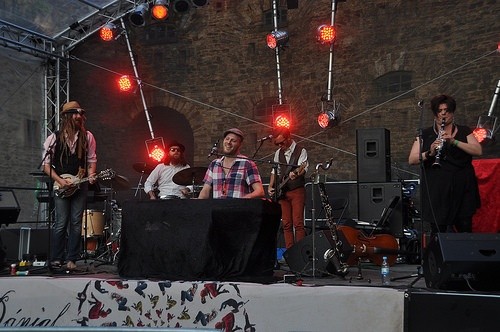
[[224, 128, 244, 142], [60, 101, 86, 114]]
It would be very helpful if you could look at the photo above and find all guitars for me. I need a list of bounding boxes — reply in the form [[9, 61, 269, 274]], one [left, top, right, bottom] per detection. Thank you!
[[271, 161, 308, 199], [53, 168, 116, 199]]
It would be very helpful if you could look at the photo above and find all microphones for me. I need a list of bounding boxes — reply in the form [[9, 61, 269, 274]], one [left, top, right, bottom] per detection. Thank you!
[[257, 135, 273, 142], [418, 99, 424, 108], [207, 147, 217, 159]]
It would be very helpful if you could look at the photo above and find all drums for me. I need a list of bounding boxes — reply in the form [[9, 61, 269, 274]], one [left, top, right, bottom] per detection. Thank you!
[[80, 209, 109, 239]]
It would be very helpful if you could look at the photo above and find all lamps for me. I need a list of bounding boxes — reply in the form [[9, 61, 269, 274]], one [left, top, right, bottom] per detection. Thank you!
[[100, 23, 126, 42], [266, 31, 289, 50], [149, 145, 167, 164], [275, 114, 292, 129], [313, 11, 337, 46], [318, 93, 341, 129], [118, 75, 144, 94], [473, 121, 495, 146], [129, 0, 207, 28]]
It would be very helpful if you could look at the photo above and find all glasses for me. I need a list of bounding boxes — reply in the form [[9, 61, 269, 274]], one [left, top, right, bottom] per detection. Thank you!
[[274, 137, 289, 146], [169, 148, 180, 152]]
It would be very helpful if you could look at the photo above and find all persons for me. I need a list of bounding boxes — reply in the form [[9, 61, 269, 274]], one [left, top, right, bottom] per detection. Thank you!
[[198, 128, 265, 199], [42, 101, 97, 269], [144, 142, 193, 199], [269, 126, 308, 264], [408, 95, 482, 233]]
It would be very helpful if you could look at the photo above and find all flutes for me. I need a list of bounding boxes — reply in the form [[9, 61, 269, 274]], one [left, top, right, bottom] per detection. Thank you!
[[432, 117, 446, 169]]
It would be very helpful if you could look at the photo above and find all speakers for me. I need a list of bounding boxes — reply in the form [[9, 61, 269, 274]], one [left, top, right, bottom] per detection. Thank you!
[[404, 288, 500, 332], [356, 128, 390, 183], [358, 183, 403, 238], [0, 190, 21, 225], [282, 229, 351, 277], [422, 232, 500, 294], [305, 181, 357, 229]]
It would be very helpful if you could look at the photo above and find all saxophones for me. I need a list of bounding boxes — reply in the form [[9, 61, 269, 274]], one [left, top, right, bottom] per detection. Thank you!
[[315, 157, 350, 276]]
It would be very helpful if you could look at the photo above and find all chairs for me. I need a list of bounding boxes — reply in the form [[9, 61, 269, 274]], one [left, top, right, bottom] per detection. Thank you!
[[310, 199, 349, 234], [369, 196, 400, 237]]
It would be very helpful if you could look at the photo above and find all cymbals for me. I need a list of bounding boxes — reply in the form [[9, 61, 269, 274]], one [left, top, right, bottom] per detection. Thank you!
[[172, 167, 208, 186], [99, 174, 130, 192]]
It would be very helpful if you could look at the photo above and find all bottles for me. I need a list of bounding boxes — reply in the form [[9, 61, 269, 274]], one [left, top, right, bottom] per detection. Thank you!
[[381, 257, 390, 286], [11, 264, 16, 275]]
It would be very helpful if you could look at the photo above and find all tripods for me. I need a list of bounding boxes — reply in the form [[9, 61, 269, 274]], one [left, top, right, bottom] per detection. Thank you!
[[60, 142, 119, 268], [255, 153, 300, 275], [386, 108, 424, 289]]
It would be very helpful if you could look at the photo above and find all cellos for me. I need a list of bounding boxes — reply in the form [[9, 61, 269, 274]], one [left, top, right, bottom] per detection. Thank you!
[[335, 225, 423, 266]]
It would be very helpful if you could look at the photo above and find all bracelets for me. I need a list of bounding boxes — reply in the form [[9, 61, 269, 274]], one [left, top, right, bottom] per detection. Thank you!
[[453, 140, 459, 146]]
